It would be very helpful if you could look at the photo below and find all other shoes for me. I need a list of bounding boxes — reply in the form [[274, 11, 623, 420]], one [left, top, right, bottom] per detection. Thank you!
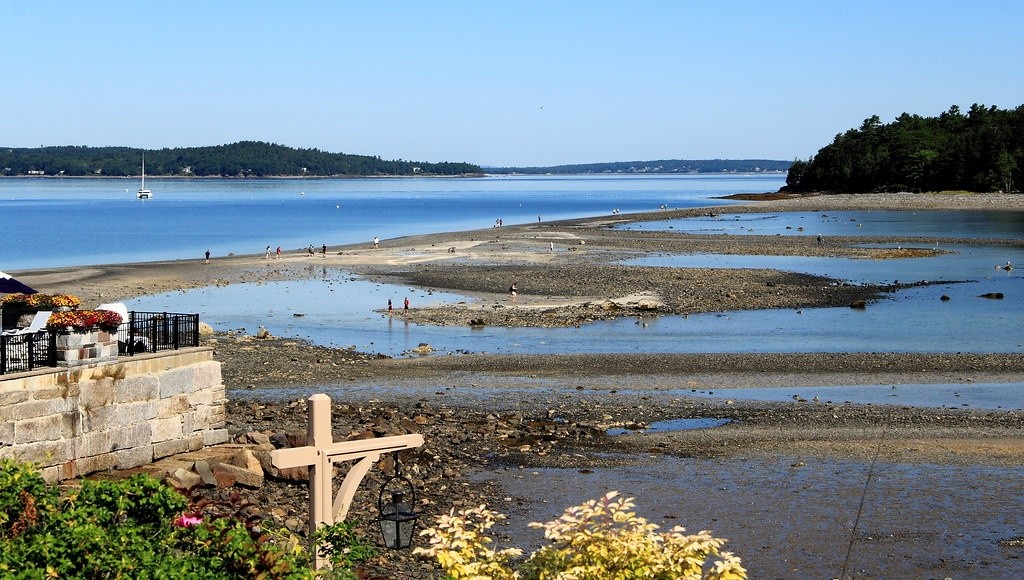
[[208, 263, 210, 264], [205, 263, 207, 264]]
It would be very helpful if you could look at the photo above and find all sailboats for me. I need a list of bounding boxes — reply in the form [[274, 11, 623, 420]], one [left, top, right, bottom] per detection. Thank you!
[[136, 152, 152, 198]]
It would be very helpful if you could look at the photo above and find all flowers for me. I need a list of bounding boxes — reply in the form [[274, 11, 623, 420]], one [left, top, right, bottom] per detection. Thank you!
[[0, 292, 81, 312], [50, 310, 123, 335]]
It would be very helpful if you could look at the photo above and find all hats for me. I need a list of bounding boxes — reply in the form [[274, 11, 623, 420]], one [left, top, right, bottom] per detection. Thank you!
[[404, 296, 408, 299]]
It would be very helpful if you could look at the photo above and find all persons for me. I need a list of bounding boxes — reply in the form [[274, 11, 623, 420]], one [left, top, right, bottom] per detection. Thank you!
[[307, 244, 312, 256], [322, 244, 326, 258], [404, 297, 409, 316], [388, 298, 392, 316], [550, 240, 553, 251], [205, 250, 210, 264], [374, 237, 379, 249], [538, 214, 541, 222], [613, 209, 615, 214], [493, 217, 503, 228], [310, 245, 315, 257], [511, 284, 517, 298], [266, 246, 272, 260], [276, 247, 282, 260], [616, 208, 619, 213], [659, 203, 669, 210], [817, 234, 822, 245]]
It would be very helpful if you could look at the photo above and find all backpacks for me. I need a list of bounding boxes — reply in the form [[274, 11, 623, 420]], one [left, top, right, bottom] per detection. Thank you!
[[509, 286, 513, 292]]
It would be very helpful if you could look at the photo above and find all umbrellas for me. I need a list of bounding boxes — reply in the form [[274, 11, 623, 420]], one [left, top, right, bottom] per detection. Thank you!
[[0, 271, 39, 295]]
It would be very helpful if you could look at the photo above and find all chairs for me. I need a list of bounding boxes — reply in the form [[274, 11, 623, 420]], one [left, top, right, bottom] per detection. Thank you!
[[0, 310, 53, 371]]
